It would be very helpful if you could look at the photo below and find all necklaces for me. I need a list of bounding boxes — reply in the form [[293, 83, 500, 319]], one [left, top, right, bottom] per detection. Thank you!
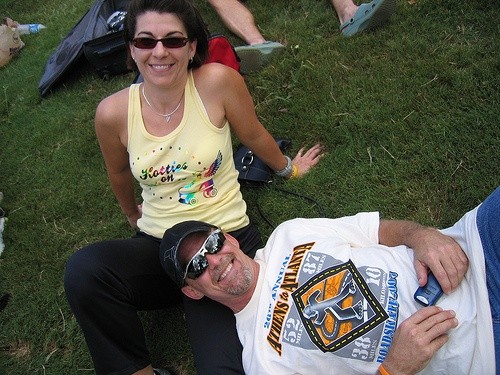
[[142, 84, 181, 122]]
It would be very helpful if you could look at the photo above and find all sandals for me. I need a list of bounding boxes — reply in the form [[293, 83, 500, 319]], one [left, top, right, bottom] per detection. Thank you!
[[339, 0, 394, 38], [234, 41, 287, 76]]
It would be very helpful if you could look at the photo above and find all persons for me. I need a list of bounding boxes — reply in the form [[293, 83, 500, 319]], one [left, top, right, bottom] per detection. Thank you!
[[63, 0, 328, 375], [157, 186, 500, 375], [207, 0, 397, 73]]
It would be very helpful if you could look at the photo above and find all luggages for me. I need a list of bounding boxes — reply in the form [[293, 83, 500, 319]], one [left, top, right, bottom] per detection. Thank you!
[[39, 0, 135, 97]]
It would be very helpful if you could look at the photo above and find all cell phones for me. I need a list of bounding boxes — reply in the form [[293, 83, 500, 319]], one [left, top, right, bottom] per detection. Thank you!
[[414, 271, 443, 307]]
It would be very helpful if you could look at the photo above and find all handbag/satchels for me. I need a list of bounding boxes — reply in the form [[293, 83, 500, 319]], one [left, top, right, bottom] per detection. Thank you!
[[233, 139, 290, 188], [0, 24, 25, 69]]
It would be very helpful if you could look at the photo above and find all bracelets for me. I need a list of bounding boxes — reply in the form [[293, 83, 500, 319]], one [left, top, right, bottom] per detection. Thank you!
[[273, 154, 293, 177], [377, 365, 390, 375], [288, 164, 298, 180]]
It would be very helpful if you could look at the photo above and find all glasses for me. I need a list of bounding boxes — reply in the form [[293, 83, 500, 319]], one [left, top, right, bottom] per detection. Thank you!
[[181, 227, 226, 287], [132, 37, 190, 49]]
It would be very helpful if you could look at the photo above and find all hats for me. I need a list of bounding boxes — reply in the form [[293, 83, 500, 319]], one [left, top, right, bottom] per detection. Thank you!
[[159, 221, 218, 287]]
